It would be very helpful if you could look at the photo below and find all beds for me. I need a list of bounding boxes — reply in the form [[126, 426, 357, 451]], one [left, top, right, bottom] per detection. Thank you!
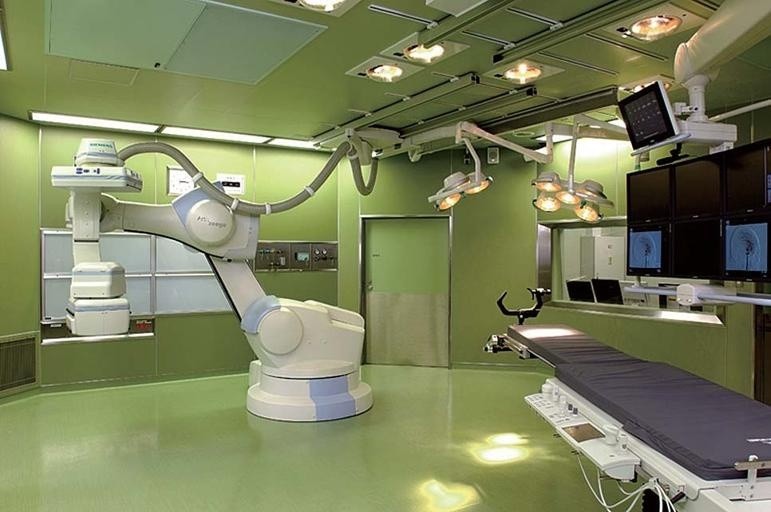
[[484, 288, 771, 512]]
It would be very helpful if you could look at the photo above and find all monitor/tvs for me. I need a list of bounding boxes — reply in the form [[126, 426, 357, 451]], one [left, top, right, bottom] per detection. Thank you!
[[618, 81, 678, 150], [627, 138, 771, 283]]
